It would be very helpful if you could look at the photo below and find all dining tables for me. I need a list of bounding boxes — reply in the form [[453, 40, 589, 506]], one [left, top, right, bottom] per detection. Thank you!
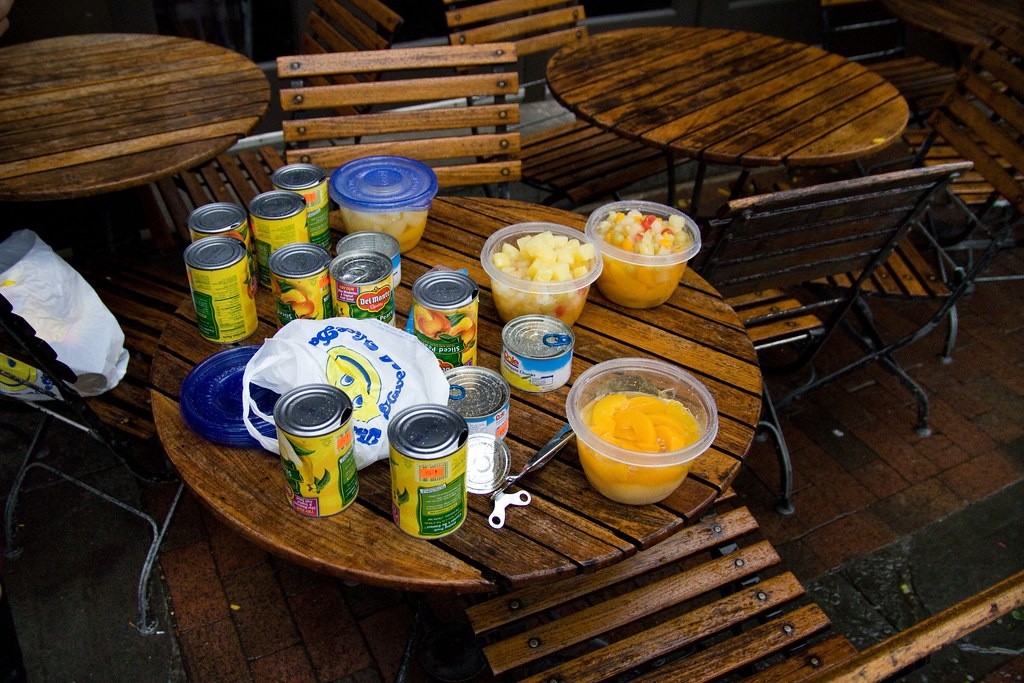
[[149, 196, 764, 683]]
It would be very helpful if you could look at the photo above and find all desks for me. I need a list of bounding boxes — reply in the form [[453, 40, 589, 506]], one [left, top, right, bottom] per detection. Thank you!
[[546, 28, 911, 255], [0, 33, 271, 282]]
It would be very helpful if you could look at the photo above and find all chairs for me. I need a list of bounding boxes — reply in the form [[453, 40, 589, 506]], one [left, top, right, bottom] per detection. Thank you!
[[0, 250, 200, 637], [155, 0, 1024, 513], [446, 485, 1024, 683]]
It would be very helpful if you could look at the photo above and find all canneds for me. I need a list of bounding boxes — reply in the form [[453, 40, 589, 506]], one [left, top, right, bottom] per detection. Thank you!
[[183, 164, 575, 540]]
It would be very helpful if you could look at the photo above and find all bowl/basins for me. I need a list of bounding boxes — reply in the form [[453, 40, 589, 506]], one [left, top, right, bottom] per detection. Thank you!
[[584, 201, 702, 310], [479, 223, 604, 328], [564, 358, 719, 504], [331, 156, 437, 255]]
